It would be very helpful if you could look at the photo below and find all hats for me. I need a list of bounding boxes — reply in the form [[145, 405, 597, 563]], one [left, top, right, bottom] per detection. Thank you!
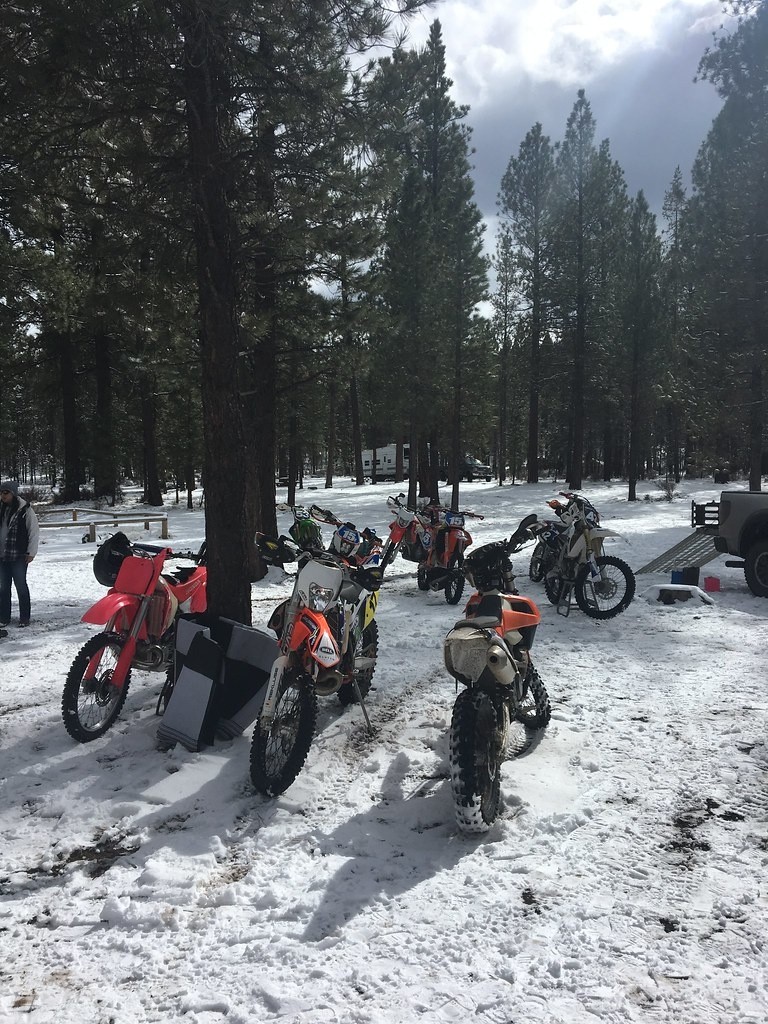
[[1, 481, 20, 498]]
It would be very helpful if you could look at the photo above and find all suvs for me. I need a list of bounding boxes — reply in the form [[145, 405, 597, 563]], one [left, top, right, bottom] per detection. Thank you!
[[465, 456, 493, 484]]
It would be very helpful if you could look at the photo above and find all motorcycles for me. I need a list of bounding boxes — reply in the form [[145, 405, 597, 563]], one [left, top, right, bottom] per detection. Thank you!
[[530, 497, 636, 620], [377, 493, 450, 577], [248, 529, 387, 800], [58, 531, 207, 742], [418, 502, 485, 606], [429, 512, 551, 834], [308, 505, 386, 589], [283, 502, 328, 551]]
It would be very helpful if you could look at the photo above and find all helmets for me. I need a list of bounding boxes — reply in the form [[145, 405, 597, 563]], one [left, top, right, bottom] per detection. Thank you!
[[93, 531, 133, 587]]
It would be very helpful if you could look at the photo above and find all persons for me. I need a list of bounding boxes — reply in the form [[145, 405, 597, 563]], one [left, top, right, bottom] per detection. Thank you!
[[0, 478, 40, 628], [0, 629, 8, 637]]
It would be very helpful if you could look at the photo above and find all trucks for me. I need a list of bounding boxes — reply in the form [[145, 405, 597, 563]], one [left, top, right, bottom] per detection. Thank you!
[[363, 443, 413, 482]]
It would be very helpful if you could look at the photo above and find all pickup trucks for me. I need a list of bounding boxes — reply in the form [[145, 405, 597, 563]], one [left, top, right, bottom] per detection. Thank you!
[[689, 490, 768, 598]]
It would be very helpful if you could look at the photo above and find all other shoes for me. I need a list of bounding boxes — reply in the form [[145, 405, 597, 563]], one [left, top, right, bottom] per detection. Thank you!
[[0, 617, 11, 627], [17, 618, 29, 627]]
